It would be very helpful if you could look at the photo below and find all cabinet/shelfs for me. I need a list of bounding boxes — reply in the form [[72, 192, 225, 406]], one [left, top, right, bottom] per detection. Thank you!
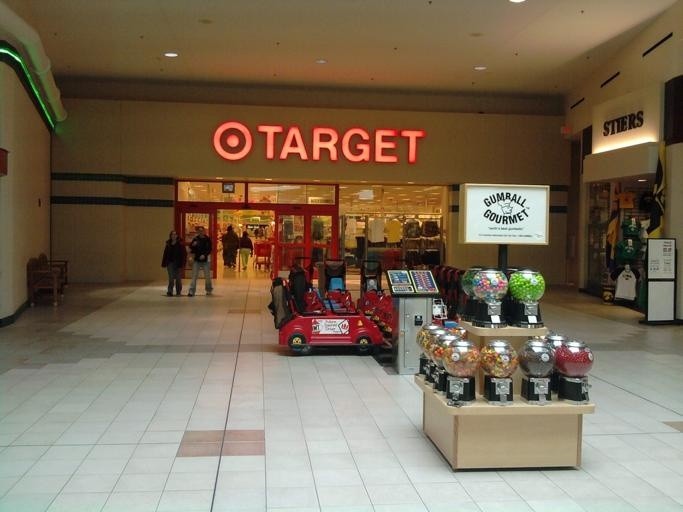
[[411, 321, 594, 472]]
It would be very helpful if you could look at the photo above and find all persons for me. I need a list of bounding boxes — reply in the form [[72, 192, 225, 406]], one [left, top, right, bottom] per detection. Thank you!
[[221, 225, 238, 268], [186, 225, 213, 296], [159, 230, 188, 296], [237, 231, 252, 272]]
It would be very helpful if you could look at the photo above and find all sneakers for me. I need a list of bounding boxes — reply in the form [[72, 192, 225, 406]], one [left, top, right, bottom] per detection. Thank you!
[[187, 291, 196, 296], [206, 290, 211, 294], [164, 291, 172, 296], [176, 292, 182, 296]]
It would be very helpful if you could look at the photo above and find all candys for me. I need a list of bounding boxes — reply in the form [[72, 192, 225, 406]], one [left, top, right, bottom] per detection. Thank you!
[[462, 271, 545, 301], [417, 328, 592, 377]]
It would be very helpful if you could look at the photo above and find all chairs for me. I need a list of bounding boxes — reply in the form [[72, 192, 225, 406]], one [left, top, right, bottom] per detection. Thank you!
[[24, 251, 69, 305]]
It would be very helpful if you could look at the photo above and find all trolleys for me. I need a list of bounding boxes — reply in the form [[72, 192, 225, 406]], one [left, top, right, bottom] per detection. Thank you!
[[252, 237, 272, 270]]
